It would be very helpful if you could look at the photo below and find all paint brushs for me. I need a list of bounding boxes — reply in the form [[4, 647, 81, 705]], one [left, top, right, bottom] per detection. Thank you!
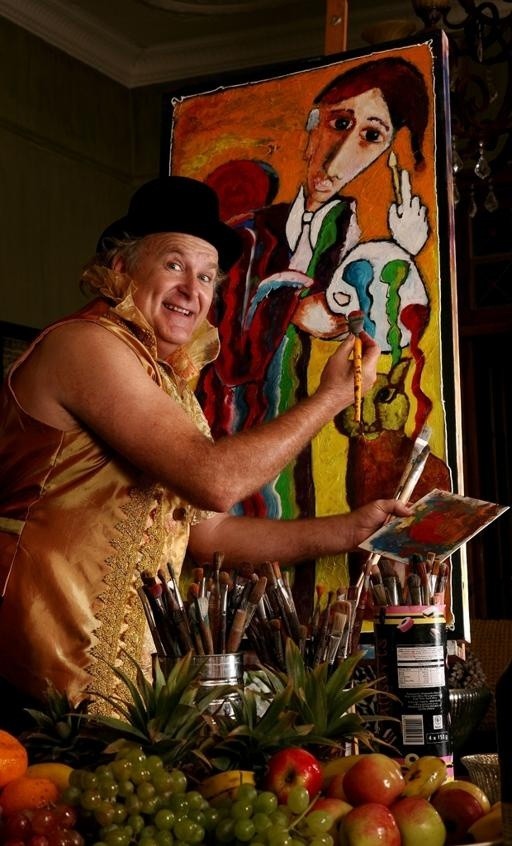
[[355, 428, 433, 608], [136, 551, 267, 654], [347, 311, 365, 423], [357, 551, 449, 606], [267, 560, 356, 682]]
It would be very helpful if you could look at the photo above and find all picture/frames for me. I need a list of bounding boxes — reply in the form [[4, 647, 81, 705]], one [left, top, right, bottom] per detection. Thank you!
[[157, 27, 474, 657]]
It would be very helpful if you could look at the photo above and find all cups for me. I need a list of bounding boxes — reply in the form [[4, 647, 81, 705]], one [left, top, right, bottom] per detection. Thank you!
[[459, 754, 499, 808]]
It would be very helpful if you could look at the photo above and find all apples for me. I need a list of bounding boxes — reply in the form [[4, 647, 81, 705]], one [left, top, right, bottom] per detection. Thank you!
[[271, 749, 489, 845]]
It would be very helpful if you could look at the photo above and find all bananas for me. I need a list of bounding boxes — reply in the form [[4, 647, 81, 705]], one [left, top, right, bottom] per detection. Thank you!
[[466, 799, 502, 841]]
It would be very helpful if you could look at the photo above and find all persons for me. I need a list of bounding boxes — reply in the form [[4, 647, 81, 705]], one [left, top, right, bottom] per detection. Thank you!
[[0, 178, 416, 733]]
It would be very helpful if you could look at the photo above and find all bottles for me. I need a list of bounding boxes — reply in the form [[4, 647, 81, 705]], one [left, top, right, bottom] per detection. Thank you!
[[353, 640, 379, 732]]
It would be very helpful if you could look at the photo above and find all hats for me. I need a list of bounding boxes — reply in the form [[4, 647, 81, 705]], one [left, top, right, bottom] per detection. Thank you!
[[96, 176, 246, 278]]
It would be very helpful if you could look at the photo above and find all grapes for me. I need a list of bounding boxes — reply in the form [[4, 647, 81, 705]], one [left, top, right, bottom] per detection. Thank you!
[[0, 803, 83, 845], [66, 747, 333, 845]]
